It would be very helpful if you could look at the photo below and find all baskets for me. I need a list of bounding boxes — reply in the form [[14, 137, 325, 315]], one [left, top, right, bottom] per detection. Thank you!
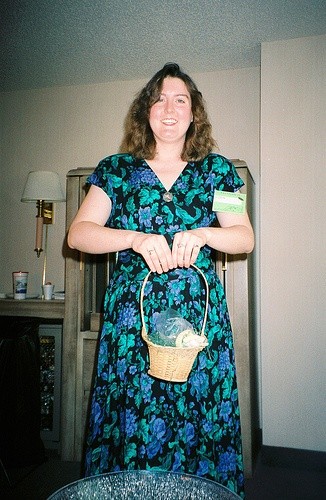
[[141, 262, 209, 383]]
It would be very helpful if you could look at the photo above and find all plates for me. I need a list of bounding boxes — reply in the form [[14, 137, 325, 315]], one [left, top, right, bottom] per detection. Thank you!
[[47, 470, 243, 500]]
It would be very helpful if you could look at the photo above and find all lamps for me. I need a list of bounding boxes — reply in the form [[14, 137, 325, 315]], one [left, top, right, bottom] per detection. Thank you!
[[21, 170, 66, 258]]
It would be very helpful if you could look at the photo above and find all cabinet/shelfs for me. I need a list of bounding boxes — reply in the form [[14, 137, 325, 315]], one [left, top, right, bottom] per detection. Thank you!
[[61, 159, 254, 478]]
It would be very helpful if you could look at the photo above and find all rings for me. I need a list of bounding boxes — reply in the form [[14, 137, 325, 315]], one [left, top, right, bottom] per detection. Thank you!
[[192, 245, 200, 250], [149, 249, 155, 254], [178, 243, 185, 248]]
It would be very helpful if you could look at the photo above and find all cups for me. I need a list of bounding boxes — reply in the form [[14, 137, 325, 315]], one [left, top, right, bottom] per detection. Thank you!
[[12, 272, 29, 300], [42, 283, 54, 300]]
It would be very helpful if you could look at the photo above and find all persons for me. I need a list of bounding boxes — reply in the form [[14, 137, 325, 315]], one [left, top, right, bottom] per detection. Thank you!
[[66, 62, 253, 498]]
